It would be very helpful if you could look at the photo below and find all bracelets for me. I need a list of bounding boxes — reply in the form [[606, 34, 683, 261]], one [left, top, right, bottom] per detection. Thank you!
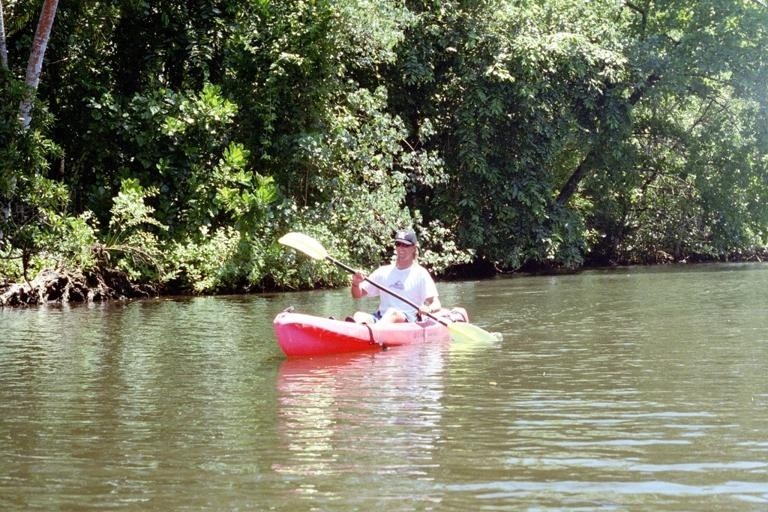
[[352, 283, 360, 288]]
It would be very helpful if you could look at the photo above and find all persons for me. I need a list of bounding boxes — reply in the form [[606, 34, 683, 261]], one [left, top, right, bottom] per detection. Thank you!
[[345, 229, 441, 323]]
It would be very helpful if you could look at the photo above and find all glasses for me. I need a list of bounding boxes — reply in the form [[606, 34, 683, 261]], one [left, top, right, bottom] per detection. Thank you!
[[394, 242, 410, 248]]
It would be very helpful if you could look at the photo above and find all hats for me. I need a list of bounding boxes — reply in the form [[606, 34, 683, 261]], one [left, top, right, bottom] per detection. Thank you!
[[390, 229, 416, 245]]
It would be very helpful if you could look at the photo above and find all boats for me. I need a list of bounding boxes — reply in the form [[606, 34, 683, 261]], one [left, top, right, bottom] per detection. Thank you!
[[273, 306, 469, 356]]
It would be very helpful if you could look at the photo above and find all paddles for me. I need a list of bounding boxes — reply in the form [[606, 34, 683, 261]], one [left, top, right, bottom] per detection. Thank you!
[[277, 231, 498, 344]]
